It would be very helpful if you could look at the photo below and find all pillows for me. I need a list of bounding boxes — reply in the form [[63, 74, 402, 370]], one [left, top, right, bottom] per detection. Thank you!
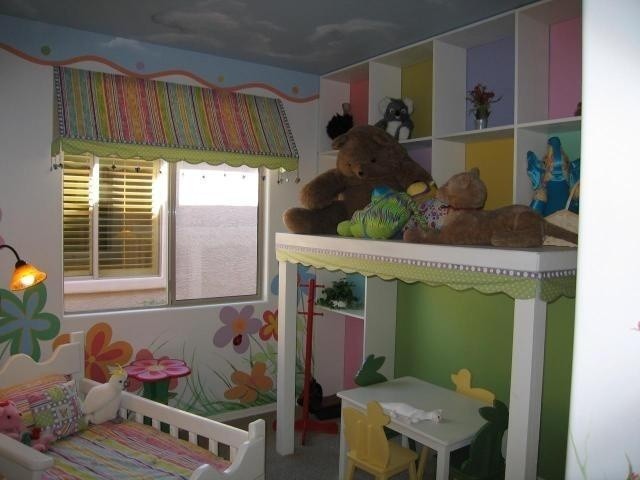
[[1, 371, 85, 445]]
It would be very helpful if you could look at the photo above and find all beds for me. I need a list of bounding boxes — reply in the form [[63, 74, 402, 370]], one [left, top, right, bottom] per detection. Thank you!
[[1, 330, 268, 480]]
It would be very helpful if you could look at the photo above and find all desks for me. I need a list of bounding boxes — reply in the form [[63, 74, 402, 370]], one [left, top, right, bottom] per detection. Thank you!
[[124, 357, 191, 435], [336, 375, 491, 480]]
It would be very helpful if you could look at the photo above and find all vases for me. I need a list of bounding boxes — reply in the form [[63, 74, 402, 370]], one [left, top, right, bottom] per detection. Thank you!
[[476, 119, 487, 131]]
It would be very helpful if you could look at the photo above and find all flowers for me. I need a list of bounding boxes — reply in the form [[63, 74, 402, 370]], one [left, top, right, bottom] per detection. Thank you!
[[464, 82, 502, 120]]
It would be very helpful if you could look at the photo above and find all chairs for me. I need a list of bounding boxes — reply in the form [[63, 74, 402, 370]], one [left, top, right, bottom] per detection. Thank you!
[[424, 399, 510, 480], [418, 369, 496, 480], [341, 401, 420, 479], [353, 354, 418, 473]]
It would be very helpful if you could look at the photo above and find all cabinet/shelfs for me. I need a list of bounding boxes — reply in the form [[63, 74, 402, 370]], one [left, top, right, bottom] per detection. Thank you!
[[310, 266, 399, 399], [318, 0, 582, 244]]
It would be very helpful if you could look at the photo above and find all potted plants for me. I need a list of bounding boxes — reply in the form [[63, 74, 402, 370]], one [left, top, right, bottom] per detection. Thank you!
[[315, 277, 360, 312]]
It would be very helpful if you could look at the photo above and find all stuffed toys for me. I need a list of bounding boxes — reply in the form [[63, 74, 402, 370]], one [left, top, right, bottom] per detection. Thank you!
[[81, 364, 128, 426], [336, 186, 427, 238], [282, 123, 437, 234], [0, 400, 56, 452], [375, 97, 414, 142], [403, 168, 578, 247], [526, 137, 580, 217], [384, 402, 443, 426]]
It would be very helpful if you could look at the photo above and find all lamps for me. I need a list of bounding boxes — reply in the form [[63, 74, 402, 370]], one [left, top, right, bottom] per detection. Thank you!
[[0, 245, 46, 292]]
